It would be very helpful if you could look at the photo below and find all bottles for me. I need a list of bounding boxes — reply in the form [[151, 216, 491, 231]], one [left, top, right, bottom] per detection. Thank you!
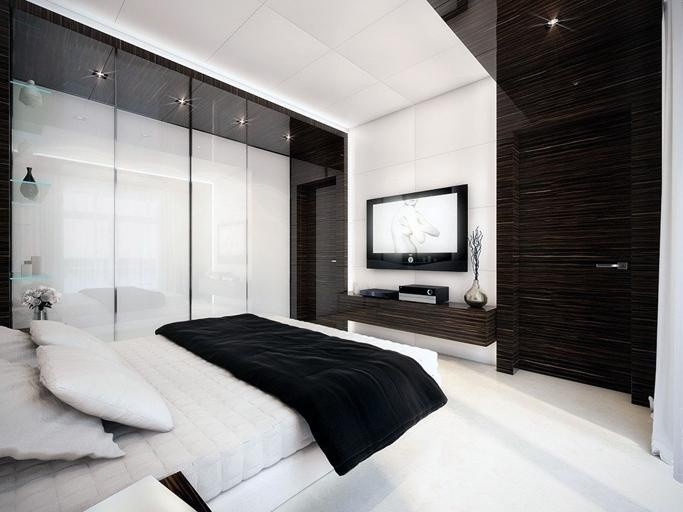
[[19, 167, 38, 200]]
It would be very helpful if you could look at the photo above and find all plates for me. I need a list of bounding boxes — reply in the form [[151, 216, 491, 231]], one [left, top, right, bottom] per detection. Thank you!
[[359, 288, 398, 298]]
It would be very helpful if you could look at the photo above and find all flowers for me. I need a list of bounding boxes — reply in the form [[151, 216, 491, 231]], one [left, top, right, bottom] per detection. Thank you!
[[21, 286, 60, 310], [29, 308, 48, 321]]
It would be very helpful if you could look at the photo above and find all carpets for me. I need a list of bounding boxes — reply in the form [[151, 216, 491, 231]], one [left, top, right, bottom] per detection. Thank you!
[[373, 354, 682, 512]]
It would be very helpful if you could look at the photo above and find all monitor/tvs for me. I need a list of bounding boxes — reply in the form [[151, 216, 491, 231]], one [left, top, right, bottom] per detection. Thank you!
[[365, 184, 469, 272]]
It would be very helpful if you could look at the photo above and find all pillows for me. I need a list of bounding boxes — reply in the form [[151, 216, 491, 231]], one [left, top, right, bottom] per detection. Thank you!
[[0, 318, 173, 462]]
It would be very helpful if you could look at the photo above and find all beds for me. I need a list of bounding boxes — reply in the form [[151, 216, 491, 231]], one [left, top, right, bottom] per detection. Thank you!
[[0, 313, 443, 512]]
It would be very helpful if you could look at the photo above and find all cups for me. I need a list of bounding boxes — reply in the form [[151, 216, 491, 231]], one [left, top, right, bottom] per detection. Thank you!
[[20, 255, 43, 275]]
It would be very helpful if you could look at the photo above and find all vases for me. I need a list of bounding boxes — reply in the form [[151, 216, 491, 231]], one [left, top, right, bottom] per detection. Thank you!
[[464, 280, 487, 307]]
